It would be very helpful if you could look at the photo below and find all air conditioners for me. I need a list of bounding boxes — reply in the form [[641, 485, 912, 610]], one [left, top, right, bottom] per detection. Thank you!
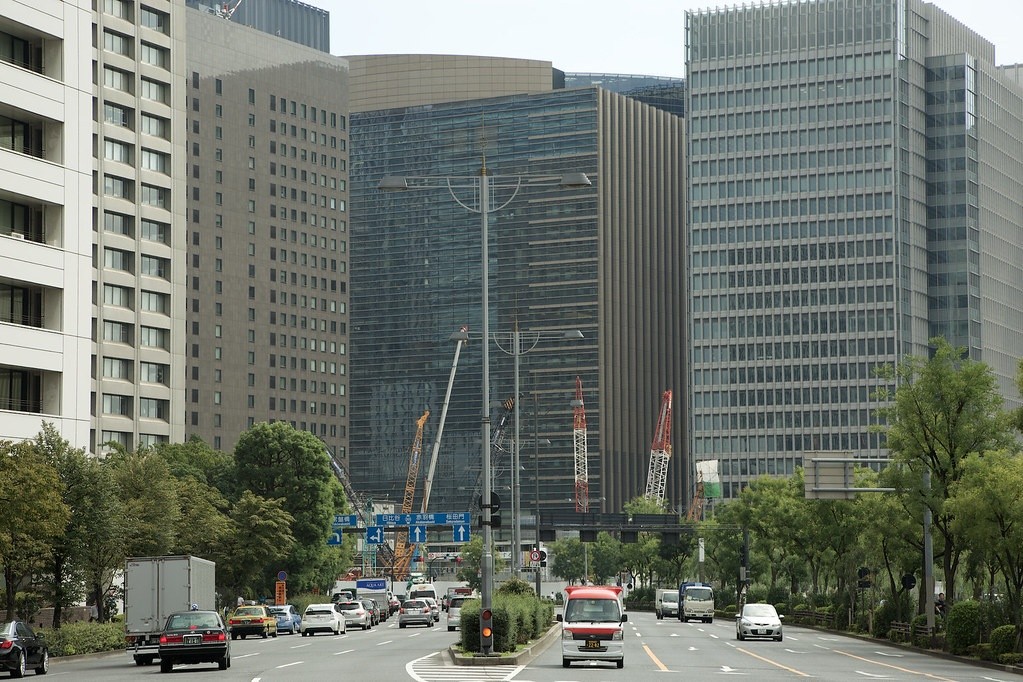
[[6, 232, 25, 240]]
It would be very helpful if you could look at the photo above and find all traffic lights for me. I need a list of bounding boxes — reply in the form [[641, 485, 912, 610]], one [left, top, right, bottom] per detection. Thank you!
[[451, 559, 461, 562], [413, 558, 424, 562]]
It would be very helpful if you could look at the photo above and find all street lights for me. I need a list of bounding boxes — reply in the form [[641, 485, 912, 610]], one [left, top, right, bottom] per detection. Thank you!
[[375, 172, 594, 648], [449, 329, 585, 590], [457, 436, 551, 585]]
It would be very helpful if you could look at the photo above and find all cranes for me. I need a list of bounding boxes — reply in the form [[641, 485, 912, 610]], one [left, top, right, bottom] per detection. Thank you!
[[464, 390, 525, 535], [573, 376, 589, 513], [645, 389, 675, 516], [306, 410, 430, 580]]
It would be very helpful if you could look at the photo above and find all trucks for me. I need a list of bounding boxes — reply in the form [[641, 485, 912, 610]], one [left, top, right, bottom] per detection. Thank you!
[[556, 585, 628, 668], [678, 581, 715, 623], [655, 589, 680, 620], [124, 555, 229, 666]]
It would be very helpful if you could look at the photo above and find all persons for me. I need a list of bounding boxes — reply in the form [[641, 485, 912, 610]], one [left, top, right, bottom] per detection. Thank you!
[[224, 595, 270, 615], [935, 592, 946, 617], [570, 600, 591, 620]]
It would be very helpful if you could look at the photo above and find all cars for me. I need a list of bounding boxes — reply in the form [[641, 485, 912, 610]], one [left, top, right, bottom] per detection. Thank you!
[[269, 604, 302, 634], [0, 620, 50, 679], [158, 604, 231, 672], [965, 593, 1006, 603], [734, 603, 785, 642], [300, 577, 477, 637], [229, 601, 278, 640]]
